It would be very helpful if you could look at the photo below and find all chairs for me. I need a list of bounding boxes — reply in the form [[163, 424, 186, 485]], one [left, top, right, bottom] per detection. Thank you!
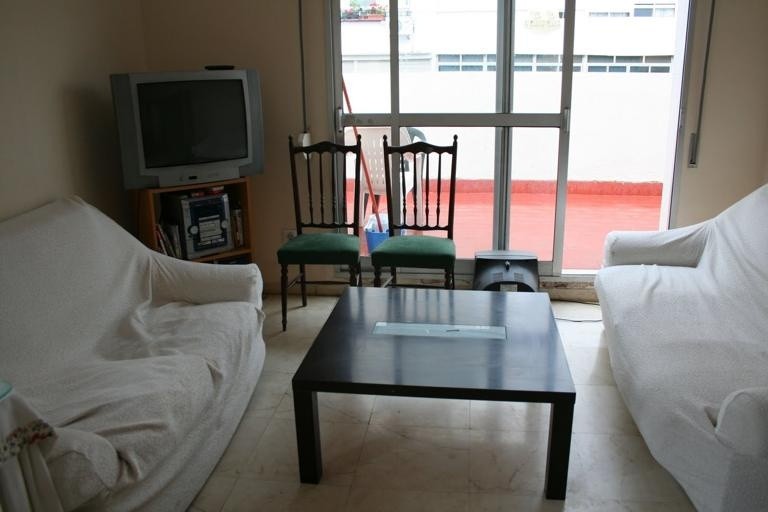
[[371, 135, 458, 287], [343, 127, 425, 236], [278, 134, 362, 331]]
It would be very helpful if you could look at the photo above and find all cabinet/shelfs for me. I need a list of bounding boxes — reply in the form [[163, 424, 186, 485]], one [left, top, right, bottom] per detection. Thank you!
[[131, 177, 255, 266]]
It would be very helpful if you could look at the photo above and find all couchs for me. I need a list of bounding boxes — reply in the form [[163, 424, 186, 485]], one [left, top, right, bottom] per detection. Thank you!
[[593, 182, 768, 511], [0, 194, 263, 512]]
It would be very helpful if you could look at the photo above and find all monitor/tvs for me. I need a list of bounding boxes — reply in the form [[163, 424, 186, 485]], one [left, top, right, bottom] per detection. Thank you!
[[108, 69, 265, 191]]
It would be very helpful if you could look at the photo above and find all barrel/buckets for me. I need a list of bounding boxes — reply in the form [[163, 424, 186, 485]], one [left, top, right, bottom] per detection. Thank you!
[[365, 212, 404, 253]]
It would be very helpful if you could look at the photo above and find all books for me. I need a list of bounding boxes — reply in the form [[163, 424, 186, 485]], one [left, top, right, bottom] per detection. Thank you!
[[231, 210, 244, 248], [156, 224, 182, 258]]
